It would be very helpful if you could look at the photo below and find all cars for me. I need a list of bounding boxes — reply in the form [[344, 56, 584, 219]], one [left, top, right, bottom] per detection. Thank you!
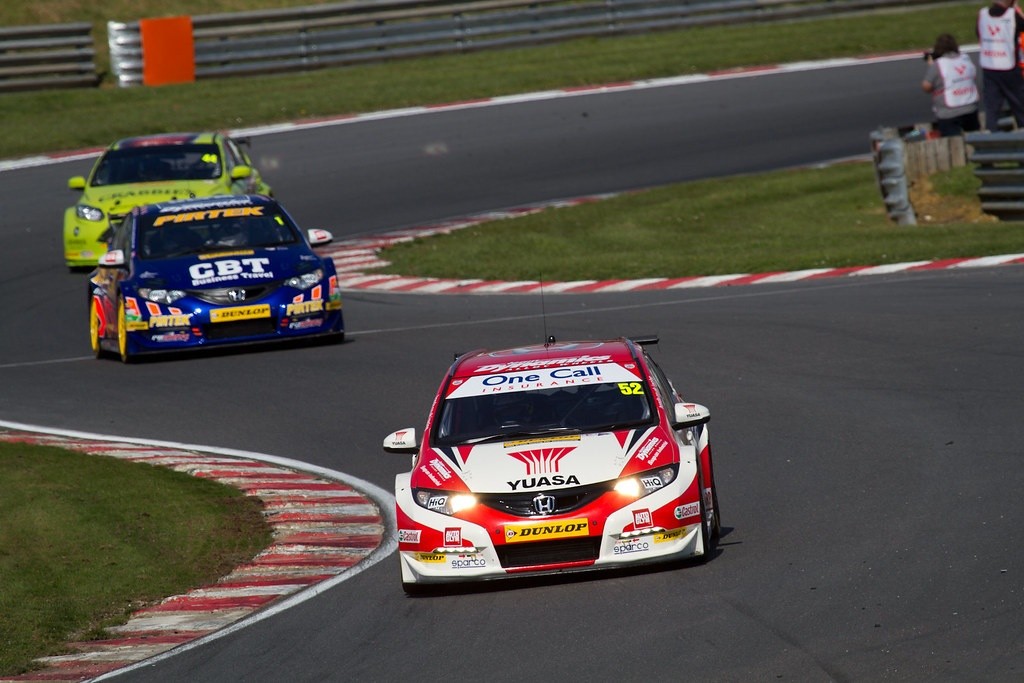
[[88, 191, 348, 362], [377, 269, 725, 592], [63, 130, 272, 273]]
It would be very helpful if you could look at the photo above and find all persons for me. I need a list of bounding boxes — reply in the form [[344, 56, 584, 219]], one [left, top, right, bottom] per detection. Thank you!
[[976, 0, 1024, 132], [922, 34, 981, 138]]
